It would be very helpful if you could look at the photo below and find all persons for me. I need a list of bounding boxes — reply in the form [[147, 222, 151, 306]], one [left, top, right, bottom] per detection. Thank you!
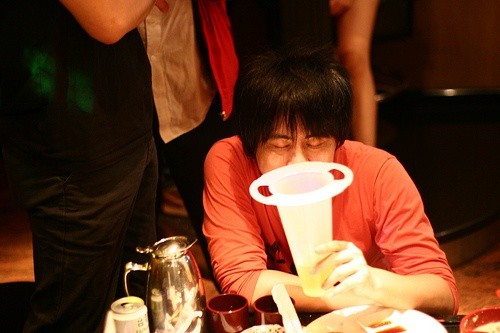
[[234, 1, 380, 149], [201, 52, 459, 316], [134, 0, 235, 295], [0, 0, 170, 333]]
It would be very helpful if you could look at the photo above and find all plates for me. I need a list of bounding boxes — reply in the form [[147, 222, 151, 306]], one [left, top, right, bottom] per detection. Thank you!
[[302, 305, 448, 333]]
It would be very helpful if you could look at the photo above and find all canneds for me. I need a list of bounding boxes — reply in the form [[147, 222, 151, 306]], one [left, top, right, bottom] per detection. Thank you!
[[111, 297, 149, 332]]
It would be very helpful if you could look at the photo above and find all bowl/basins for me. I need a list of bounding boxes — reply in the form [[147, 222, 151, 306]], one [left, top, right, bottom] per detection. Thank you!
[[460, 304, 500, 333]]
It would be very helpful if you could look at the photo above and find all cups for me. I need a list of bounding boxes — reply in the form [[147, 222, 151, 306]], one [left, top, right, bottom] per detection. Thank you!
[[251, 162, 353, 298], [204, 290, 251, 333], [251, 295, 297, 327]]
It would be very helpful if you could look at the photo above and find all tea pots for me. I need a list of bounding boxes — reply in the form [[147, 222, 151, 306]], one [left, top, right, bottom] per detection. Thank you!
[[123, 236, 206, 333]]
